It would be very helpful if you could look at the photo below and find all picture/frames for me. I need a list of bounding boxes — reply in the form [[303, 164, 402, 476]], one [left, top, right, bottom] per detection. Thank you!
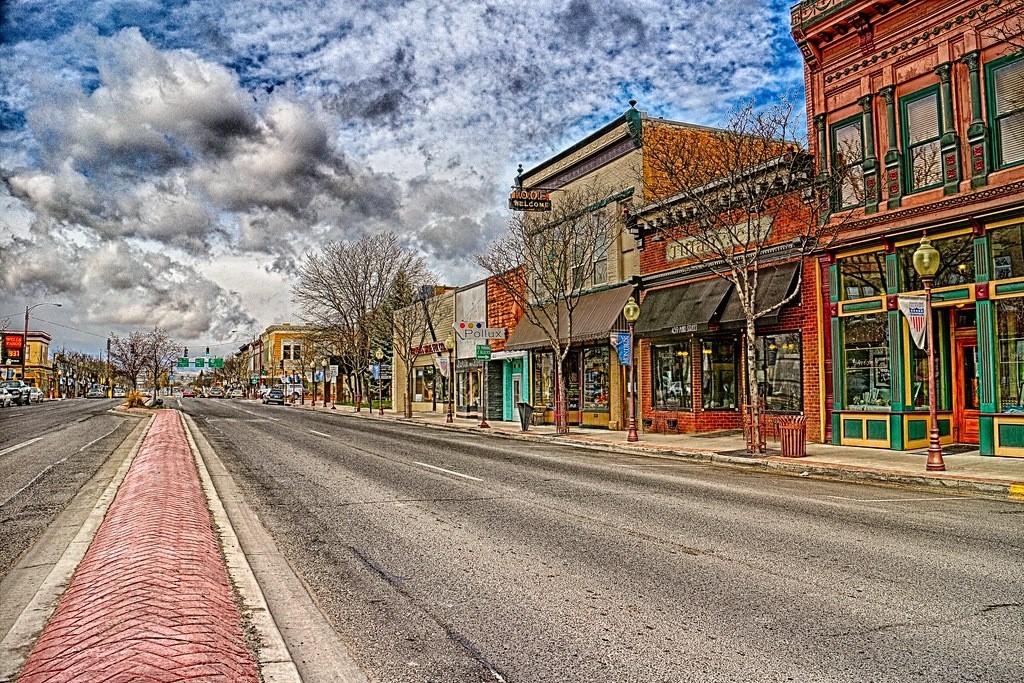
[[873, 354, 892, 388]]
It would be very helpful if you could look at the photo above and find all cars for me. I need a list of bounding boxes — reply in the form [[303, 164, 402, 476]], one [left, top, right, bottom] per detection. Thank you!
[[86, 388, 104, 398], [0, 380, 44, 408], [183, 388, 196, 398], [199, 387, 245, 398], [113, 389, 126, 398]]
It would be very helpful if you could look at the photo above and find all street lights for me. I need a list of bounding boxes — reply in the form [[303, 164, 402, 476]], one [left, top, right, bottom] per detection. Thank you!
[[310, 361, 316, 406], [21, 302, 62, 383], [375, 347, 385, 415], [321, 358, 327, 408], [445, 334, 453, 423], [624, 296, 641, 442], [913, 237, 946, 471], [231, 330, 256, 398], [292, 364, 296, 403]]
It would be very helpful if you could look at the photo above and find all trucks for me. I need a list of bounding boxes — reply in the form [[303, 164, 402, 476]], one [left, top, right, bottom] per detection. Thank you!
[[258, 383, 306, 401]]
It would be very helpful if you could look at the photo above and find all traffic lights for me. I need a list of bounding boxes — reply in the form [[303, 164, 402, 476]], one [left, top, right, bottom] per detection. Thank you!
[[260, 369, 265, 375], [184, 347, 189, 358], [205, 347, 210, 356], [280, 359, 284, 370]]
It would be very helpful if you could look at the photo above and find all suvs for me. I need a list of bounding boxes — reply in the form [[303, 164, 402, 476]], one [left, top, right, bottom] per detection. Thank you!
[[263, 388, 284, 406]]
